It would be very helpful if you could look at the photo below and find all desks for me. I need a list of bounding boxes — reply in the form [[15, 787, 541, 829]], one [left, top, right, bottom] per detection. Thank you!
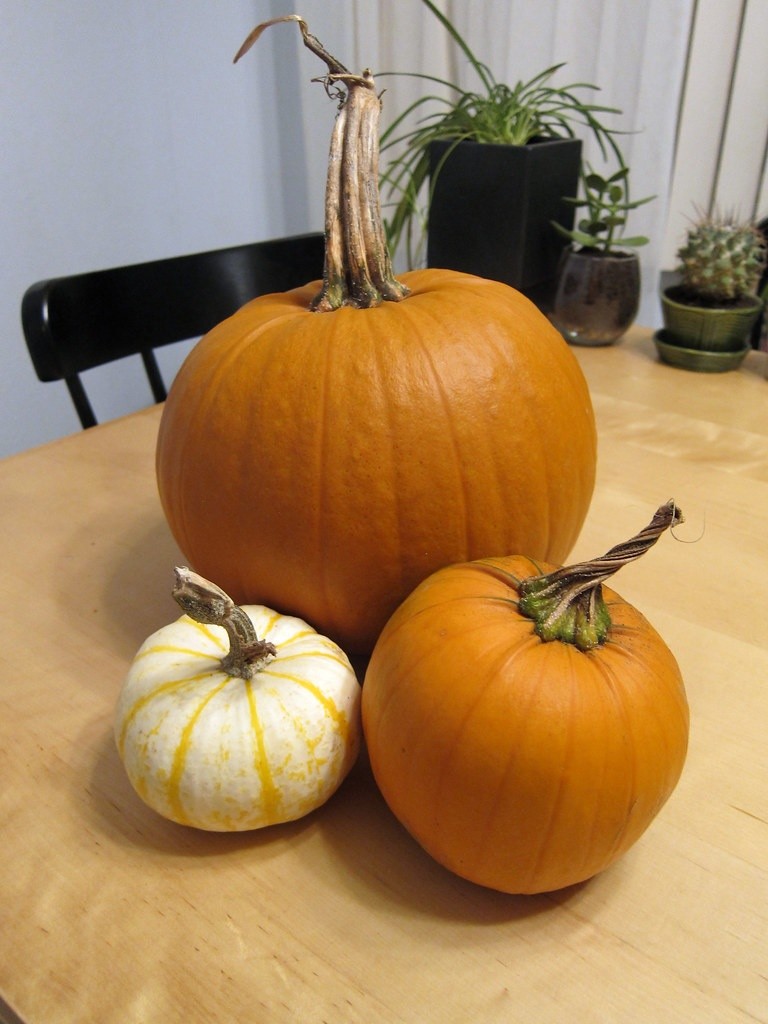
[[0, 310, 768, 1024]]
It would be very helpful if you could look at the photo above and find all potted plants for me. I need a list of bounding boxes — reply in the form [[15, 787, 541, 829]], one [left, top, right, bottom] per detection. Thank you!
[[547, 165, 660, 348], [650, 195, 768, 376], [371, 0, 649, 317]]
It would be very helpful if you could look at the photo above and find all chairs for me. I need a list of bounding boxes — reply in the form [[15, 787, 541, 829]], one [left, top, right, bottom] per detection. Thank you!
[[19, 231, 332, 430]]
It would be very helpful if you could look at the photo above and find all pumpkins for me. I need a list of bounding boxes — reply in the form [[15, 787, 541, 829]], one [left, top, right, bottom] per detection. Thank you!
[[360, 498, 690, 895], [113, 566, 364, 831], [156, 16, 599, 667]]
